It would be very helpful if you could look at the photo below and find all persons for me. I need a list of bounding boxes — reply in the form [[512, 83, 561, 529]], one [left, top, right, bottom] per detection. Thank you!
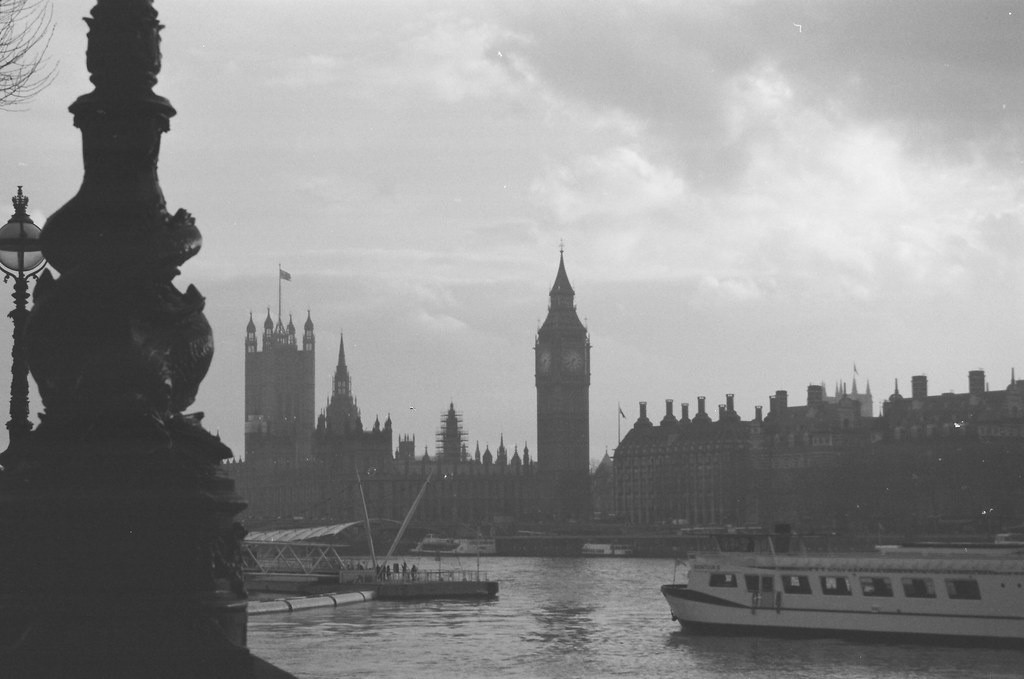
[[775, 589, 783, 615], [752, 591, 761, 616], [337, 561, 420, 581]]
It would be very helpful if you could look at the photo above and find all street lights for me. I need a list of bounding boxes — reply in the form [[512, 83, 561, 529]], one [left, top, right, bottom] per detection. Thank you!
[[0, 184, 48, 546]]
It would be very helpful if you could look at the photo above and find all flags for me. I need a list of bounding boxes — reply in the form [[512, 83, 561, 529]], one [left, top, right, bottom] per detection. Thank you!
[[279, 270, 291, 281]]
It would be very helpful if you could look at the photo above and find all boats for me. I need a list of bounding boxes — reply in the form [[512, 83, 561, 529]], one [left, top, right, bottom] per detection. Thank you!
[[372, 533, 498, 601], [410, 524, 499, 558], [659, 534, 1024, 648], [579, 540, 634, 558]]
